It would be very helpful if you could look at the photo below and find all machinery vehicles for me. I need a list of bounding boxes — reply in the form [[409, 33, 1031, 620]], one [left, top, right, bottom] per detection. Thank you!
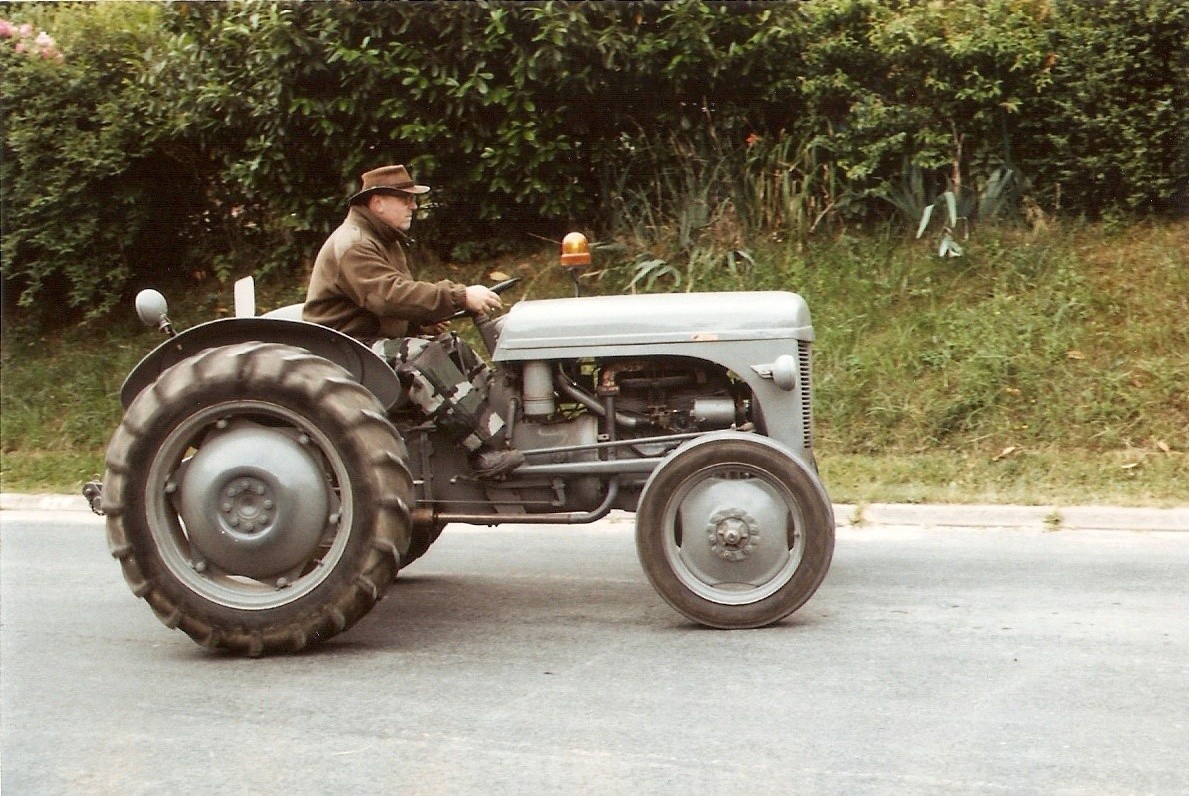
[[79, 231, 834, 653]]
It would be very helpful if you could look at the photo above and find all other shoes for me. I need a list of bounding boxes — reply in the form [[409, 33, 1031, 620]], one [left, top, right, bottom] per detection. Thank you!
[[466, 444, 525, 481]]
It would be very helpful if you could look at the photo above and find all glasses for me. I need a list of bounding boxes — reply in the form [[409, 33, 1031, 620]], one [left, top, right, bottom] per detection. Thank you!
[[372, 189, 417, 206]]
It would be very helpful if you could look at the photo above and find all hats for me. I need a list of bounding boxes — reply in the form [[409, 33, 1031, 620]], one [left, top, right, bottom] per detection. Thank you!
[[347, 164, 431, 205]]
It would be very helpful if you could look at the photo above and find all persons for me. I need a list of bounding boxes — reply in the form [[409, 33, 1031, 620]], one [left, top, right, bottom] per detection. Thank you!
[[301, 164, 524, 480]]
